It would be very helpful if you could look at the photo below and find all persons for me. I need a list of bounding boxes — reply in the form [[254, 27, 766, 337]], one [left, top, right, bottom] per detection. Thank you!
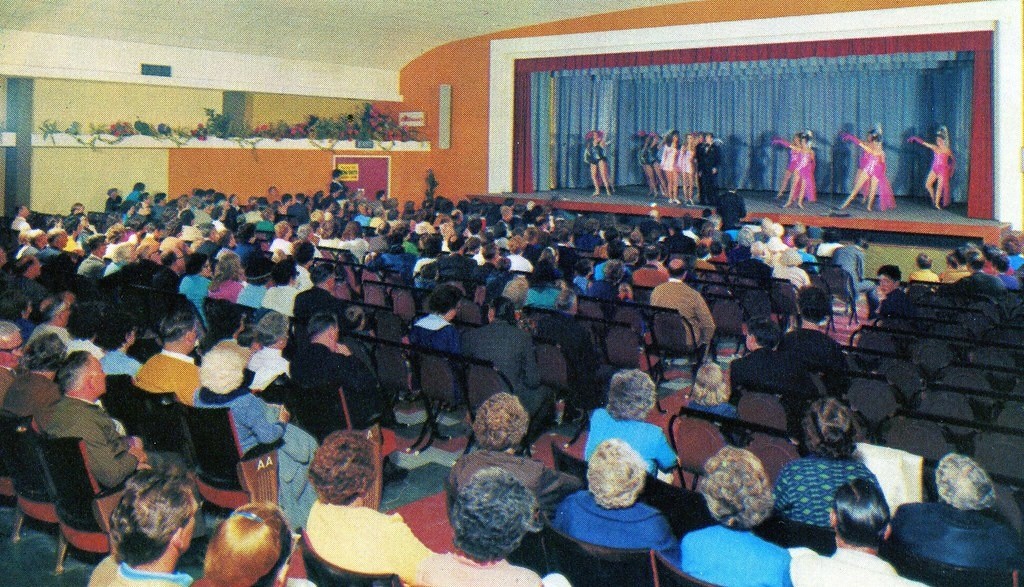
[[582, 123, 896, 210], [0, 171, 1024, 587], [909, 125, 955, 210]]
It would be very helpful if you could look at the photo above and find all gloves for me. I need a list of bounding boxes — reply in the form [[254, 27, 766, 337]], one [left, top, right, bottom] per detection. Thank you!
[[771, 139, 791, 149], [907, 136, 925, 145], [842, 134, 861, 146]]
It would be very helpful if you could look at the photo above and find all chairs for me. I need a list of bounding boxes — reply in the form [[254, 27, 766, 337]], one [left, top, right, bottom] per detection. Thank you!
[[1, 211, 1024, 587]]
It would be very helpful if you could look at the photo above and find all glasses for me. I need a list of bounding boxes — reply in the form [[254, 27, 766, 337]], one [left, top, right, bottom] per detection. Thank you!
[[203, 262, 213, 268], [453, 306, 464, 313]]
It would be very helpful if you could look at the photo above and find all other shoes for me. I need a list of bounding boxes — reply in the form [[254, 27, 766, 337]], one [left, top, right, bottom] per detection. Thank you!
[[669, 198, 679, 204], [383, 455, 408, 482], [685, 197, 694, 205]]
